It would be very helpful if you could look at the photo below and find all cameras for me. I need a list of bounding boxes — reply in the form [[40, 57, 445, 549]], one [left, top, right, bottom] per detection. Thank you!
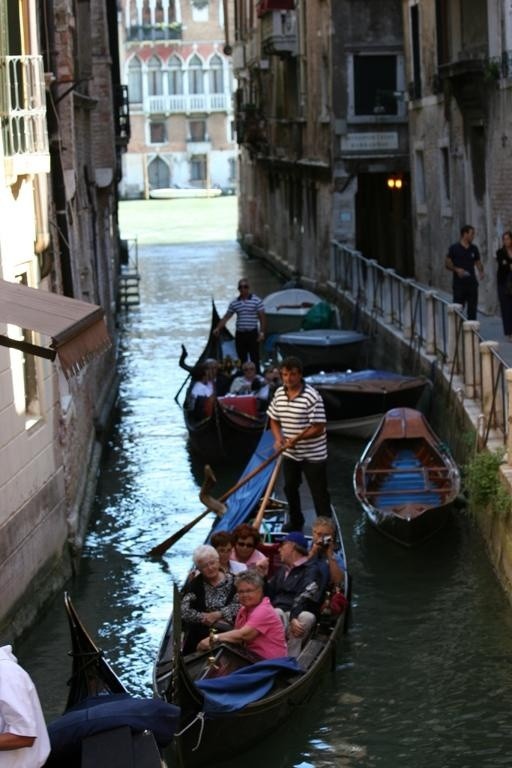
[[323, 534, 332, 546]]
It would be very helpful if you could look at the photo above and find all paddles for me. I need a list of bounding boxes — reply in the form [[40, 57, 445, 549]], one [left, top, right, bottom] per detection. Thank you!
[[147, 424, 315, 554]]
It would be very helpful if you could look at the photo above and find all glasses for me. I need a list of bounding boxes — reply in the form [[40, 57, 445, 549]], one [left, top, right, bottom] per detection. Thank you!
[[237, 284, 250, 290], [238, 542, 254, 548], [238, 587, 258, 594], [244, 367, 254, 372]]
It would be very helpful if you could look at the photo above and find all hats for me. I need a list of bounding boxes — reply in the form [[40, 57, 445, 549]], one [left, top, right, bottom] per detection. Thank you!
[[274, 531, 308, 548]]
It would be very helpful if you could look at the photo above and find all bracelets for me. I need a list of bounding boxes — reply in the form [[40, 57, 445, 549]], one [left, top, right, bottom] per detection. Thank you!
[[213, 634, 218, 643]]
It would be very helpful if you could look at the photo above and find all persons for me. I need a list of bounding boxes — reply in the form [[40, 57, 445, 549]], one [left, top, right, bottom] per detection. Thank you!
[[213, 278, 266, 375], [210, 531, 247, 576], [187, 368, 216, 409], [445, 224, 484, 322], [307, 517, 346, 589], [266, 358, 332, 532], [230, 362, 265, 395], [230, 524, 266, 566], [493, 231, 512, 343], [266, 531, 322, 660], [204, 358, 231, 396], [1, 640, 51, 768], [179, 545, 242, 655], [198, 570, 288, 670]]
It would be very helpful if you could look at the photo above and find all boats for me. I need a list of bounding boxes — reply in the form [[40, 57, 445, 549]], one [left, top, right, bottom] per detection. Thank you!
[[303, 369, 434, 442], [47, 591, 183, 767], [258, 289, 370, 374], [353, 407, 461, 547], [152, 429, 351, 766], [179, 297, 276, 462]]
